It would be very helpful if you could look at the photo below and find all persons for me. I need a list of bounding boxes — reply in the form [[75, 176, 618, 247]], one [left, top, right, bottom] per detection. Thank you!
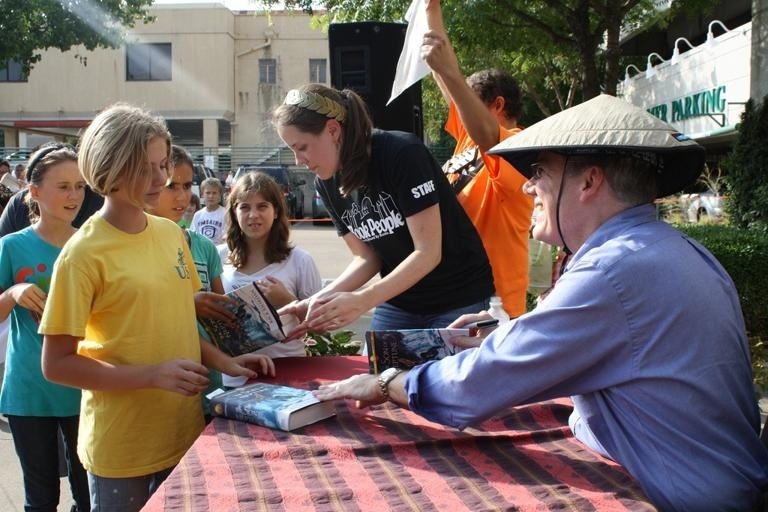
[[416, 0, 535, 323], [139, 144, 243, 425], [211, 168, 326, 391], [273, 82, 499, 358], [309, 91, 767, 512], [0, 137, 103, 481], [0, 142, 88, 512], [33, 98, 278, 512], [0, 142, 231, 250]]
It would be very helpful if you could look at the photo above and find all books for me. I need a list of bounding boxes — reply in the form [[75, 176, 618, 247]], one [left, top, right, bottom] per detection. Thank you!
[[364, 324, 468, 377], [195, 275, 286, 358], [208, 380, 337, 432]]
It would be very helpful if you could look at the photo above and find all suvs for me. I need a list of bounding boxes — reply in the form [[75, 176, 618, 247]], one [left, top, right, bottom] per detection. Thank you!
[[190, 164, 330, 220]]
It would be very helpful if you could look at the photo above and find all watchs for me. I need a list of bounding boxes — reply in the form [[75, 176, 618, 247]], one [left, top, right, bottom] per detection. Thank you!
[[376, 366, 401, 404]]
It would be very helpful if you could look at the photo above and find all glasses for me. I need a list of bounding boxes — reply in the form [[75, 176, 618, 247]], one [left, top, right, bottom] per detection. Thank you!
[[530, 162, 561, 179]]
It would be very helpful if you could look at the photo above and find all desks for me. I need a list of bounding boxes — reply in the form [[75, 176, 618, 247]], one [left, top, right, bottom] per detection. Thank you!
[[138, 357, 658, 512]]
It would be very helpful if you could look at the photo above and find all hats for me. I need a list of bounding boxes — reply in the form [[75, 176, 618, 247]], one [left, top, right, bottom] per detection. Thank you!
[[485, 94, 705, 183]]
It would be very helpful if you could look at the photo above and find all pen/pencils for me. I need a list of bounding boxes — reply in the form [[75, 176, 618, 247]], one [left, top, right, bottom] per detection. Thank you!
[[462, 319, 499, 330]]
